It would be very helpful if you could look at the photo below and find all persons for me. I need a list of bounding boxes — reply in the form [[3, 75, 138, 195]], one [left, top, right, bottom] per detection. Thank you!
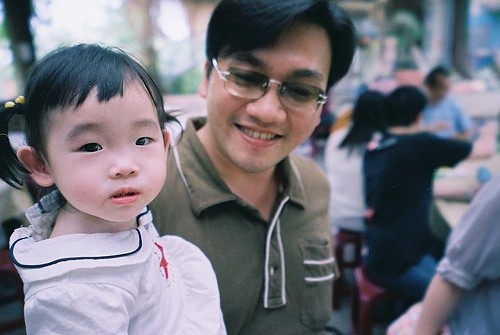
[[298, 13, 500, 324], [147, 1, 356, 335], [0, 44, 228, 335], [413, 174, 500, 335]]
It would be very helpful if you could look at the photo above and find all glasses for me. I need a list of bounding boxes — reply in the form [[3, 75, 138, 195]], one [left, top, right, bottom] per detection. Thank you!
[[210, 58, 328, 113]]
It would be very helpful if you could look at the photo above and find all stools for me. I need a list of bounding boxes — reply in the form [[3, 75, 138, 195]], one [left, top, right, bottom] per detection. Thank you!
[[333, 229, 366, 307], [350, 265, 415, 335]]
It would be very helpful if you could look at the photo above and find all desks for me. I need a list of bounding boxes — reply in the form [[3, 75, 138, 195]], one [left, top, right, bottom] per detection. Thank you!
[[457, 91, 500, 119], [430, 154, 500, 240]]
